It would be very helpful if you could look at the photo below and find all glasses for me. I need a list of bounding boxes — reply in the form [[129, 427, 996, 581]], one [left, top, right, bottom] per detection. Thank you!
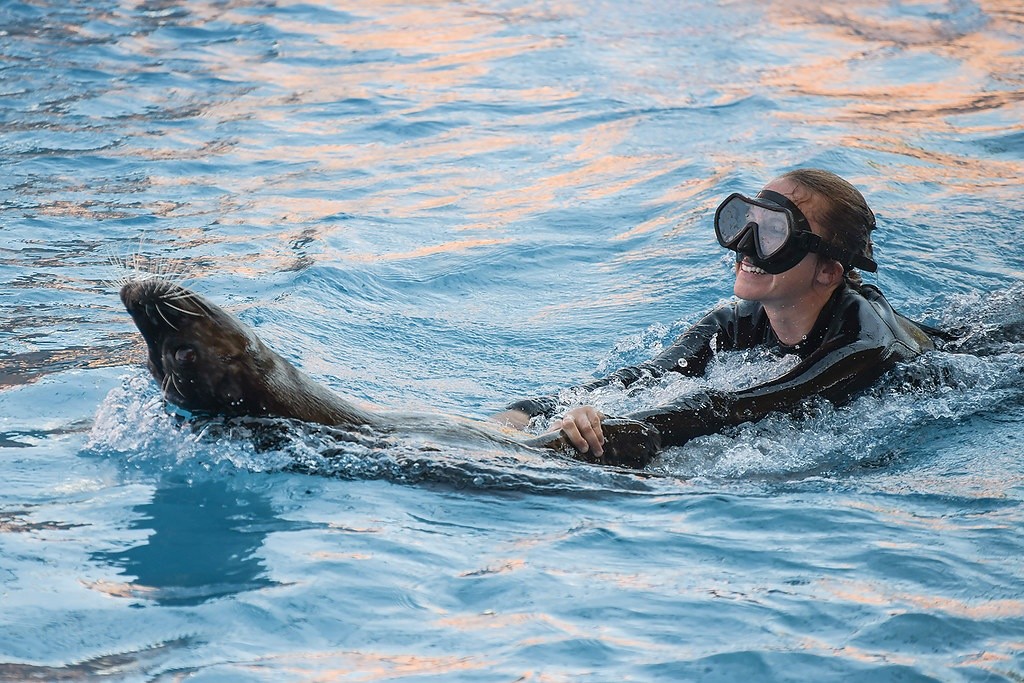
[[714, 189, 813, 274]]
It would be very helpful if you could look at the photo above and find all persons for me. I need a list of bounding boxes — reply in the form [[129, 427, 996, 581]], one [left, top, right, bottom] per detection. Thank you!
[[483, 169, 1024, 458]]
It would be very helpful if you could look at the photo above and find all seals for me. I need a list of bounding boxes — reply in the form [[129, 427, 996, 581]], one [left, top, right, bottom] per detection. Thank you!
[[90, 235, 661, 470]]
